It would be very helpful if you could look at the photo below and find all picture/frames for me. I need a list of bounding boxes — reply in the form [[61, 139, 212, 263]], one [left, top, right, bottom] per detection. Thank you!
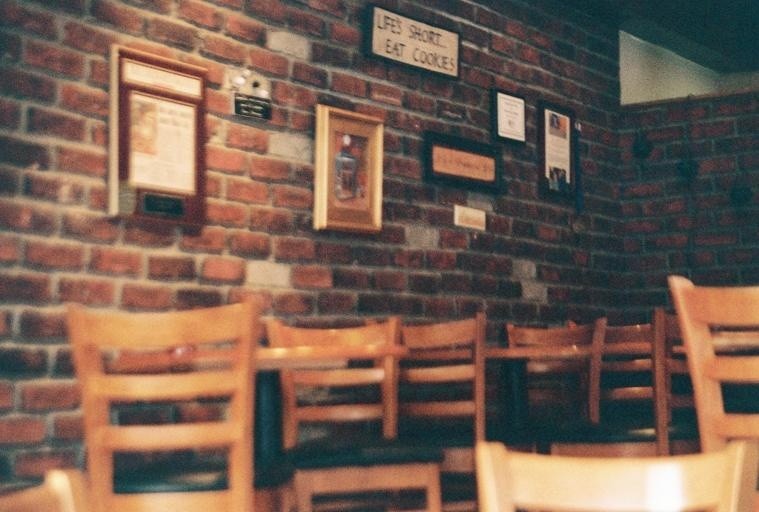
[[315, 104, 384, 234], [537, 100, 577, 203], [491, 89, 527, 147], [453, 204, 486, 232], [423, 131, 503, 193], [362, 4, 461, 80]]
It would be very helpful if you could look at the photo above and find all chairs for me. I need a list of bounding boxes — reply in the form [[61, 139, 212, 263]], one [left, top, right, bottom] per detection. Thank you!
[[0, 273, 758, 512]]
